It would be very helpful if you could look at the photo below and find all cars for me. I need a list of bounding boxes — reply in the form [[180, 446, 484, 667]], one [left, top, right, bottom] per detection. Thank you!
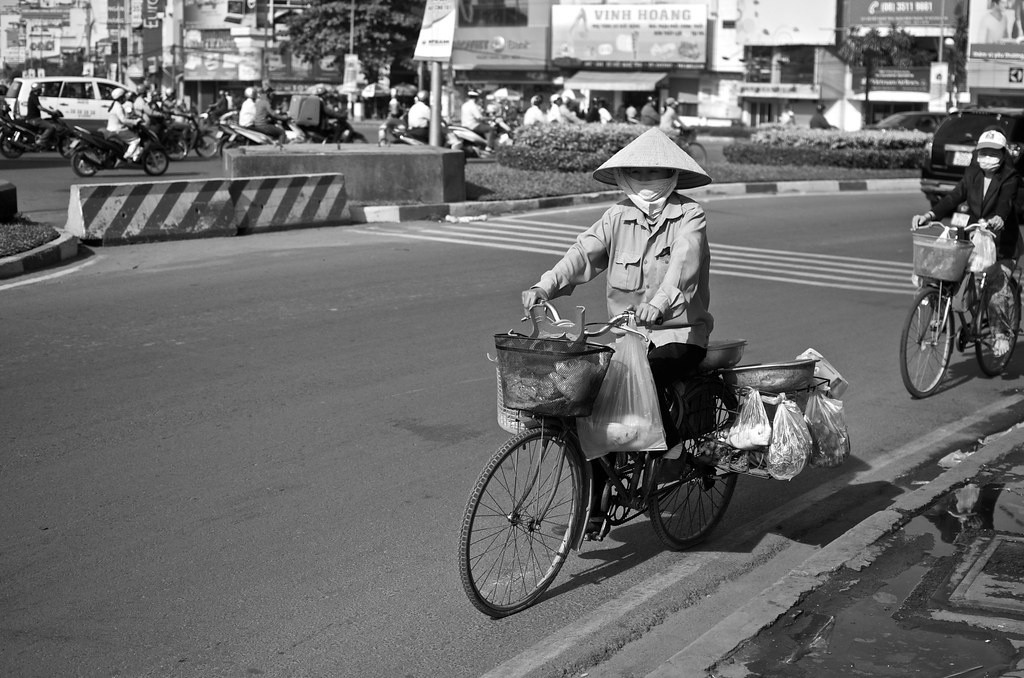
[[863, 112, 951, 135]]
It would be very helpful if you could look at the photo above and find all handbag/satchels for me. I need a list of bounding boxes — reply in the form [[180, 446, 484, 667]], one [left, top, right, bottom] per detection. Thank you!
[[580, 311, 668, 462], [952, 272, 977, 312], [969, 218, 997, 272], [726, 387, 851, 481]]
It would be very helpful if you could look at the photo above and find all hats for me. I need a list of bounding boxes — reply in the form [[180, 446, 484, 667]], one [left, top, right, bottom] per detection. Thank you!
[[311, 83, 328, 96], [976, 130, 1008, 151], [593, 126, 713, 189]]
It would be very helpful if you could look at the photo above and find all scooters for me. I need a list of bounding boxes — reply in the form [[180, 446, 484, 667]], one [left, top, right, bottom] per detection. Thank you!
[[390, 119, 451, 148], [446, 117, 513, 161]]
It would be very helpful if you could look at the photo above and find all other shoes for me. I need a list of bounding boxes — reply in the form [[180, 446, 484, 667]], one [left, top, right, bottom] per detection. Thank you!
[[552, 521, 611, 538], [655, 443, 687, 485]]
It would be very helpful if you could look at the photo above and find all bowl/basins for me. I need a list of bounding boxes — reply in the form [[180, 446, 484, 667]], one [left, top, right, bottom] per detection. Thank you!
[[700, 339, 821, 395]]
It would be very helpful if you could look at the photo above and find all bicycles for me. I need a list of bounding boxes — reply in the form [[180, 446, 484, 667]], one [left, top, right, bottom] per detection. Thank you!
[[898, 220, 1021, 399], [458, 298, 742, 618]]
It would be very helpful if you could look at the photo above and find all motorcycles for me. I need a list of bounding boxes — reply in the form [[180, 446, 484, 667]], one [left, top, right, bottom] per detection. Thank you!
[[0, 106, 78, 159], [215, 109, 306, 156], [292, 103, 368, 145], [63, 104, 169, 177], [151, 102, 217, 161]]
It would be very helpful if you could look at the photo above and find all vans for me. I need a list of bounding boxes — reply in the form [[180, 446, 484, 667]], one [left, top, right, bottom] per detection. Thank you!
[[3, 77, 150, 134]]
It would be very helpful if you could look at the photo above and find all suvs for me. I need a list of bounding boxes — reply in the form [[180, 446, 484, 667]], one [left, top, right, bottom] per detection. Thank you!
[[920, 106, 1024, 217]]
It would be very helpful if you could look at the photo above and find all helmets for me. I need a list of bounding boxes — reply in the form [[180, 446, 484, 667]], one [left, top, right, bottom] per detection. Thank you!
[[467, 89, 483, 97], [244, 87, 257, 99], [416, 90, 429, 101], [531, 94, 562, 106], [31, 82, 44, 91], [111, 84, 176, 101]]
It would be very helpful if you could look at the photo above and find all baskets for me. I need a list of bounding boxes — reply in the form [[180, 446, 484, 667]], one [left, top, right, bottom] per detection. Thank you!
[[486, 333, 615, 435], [912, 233, 975, 282]]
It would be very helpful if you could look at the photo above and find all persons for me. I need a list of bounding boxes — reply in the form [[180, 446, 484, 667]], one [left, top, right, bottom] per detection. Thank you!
[[778, 102, 796, 125], [809, 103, 836, 129], [912, 129, 1024, 358], [0, 79, 691, 160], [521, 124, 714, 538]]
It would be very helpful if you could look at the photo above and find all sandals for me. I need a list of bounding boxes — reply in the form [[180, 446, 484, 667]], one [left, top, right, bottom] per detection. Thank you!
[[992, 335, 1010, 358]]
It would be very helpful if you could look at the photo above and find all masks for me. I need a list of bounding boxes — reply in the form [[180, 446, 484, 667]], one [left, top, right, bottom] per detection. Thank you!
[[978, 156, 1004, 171]]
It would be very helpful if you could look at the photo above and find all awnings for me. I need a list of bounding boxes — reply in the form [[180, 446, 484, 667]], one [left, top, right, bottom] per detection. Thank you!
[[564, 71, 669, 91]]
